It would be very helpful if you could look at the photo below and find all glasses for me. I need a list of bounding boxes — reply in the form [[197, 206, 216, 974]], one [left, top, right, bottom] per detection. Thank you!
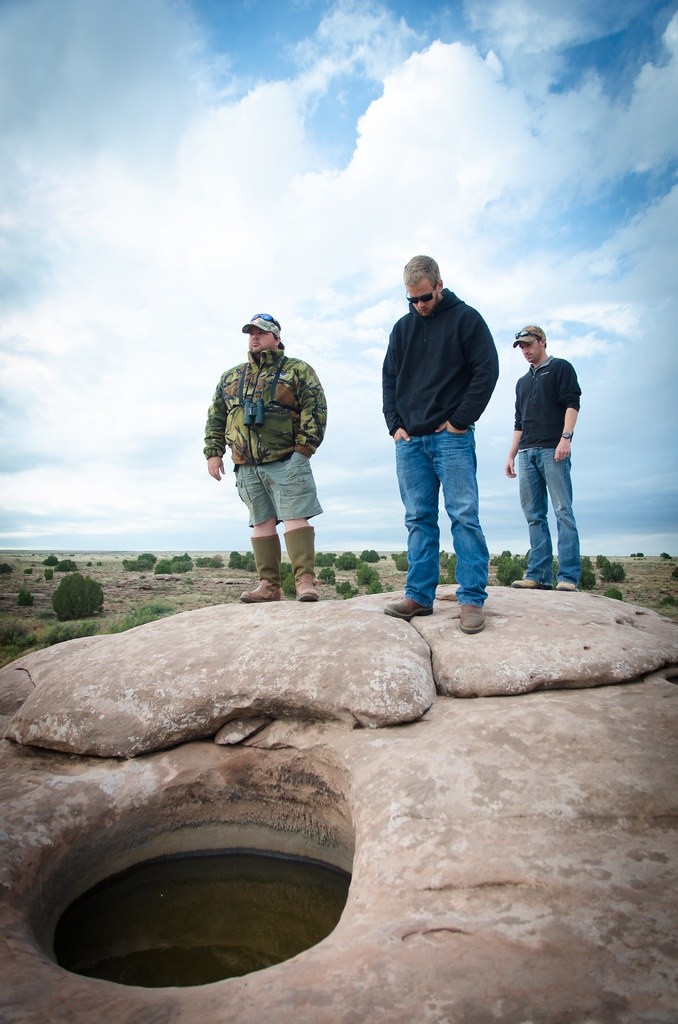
[[515, 330, 542, 340], [250, 314, 281, 331], [406, 280, 437, 304]]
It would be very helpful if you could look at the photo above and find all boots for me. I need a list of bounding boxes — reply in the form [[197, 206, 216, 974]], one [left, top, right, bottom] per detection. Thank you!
[[284, 526, 319, 601], [240, 535, 282, 603]]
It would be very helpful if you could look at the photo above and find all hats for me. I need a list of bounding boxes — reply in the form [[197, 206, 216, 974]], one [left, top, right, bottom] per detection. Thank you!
[[242, 317, 280, 339], [513, 326, 546, 348]]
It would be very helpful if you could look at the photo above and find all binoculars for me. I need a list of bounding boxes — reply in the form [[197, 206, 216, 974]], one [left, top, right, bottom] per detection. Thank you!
[[244, 398, 265, 426]]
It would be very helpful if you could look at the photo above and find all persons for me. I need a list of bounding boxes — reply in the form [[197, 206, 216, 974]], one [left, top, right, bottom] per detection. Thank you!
[[504, 326, 582, 591], [382, 255, 499, 634], [203, 314, 327, 603]]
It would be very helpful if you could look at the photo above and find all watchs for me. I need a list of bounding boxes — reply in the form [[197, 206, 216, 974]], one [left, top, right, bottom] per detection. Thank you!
[[561, 432, 571, 439]]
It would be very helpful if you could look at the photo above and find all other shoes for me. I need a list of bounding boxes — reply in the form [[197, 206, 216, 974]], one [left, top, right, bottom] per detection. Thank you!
[[460, 604, 486, 634], [384, 598, 433, 620], [512, 579, 554, 590], [555, 581, 575, 591]]
